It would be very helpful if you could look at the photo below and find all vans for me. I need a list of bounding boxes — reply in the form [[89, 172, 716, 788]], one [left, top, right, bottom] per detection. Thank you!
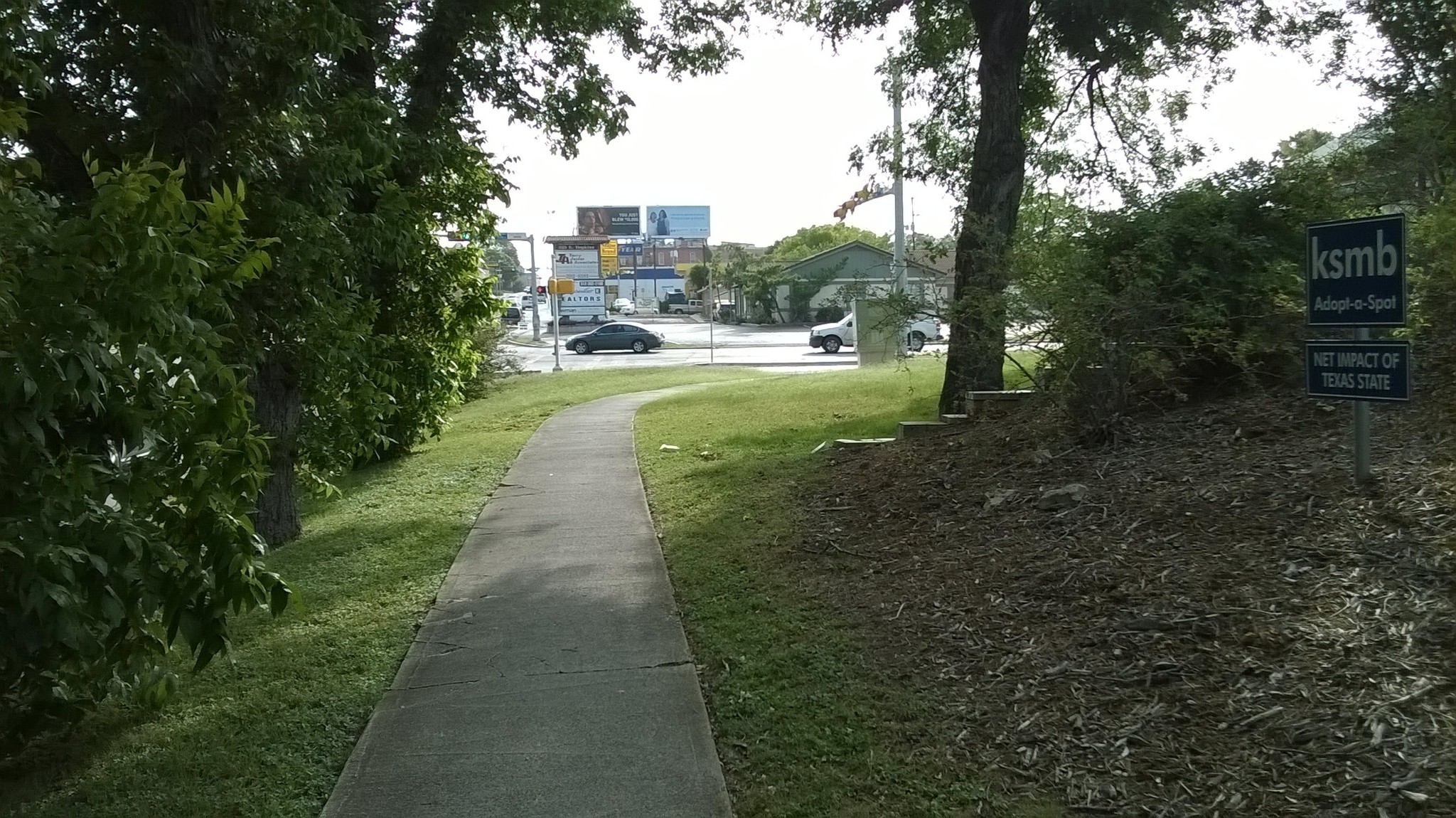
[[522, 295, 534, 310]]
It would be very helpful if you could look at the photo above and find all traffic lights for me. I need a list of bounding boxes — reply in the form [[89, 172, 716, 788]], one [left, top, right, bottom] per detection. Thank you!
[[448, 231, 472, 242], [537, 286, 543, 293]]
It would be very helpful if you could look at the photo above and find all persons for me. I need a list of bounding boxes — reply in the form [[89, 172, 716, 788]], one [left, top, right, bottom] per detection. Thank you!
[[579, 211, 603, 235], [647, 209, 670, 235]]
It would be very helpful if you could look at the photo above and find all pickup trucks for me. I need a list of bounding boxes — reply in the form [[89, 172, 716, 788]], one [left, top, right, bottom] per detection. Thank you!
[[667, 300, 704, 315], [808, 310, 944, 354]]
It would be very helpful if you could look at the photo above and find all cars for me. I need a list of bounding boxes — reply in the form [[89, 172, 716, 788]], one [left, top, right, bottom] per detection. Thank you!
[[712, 302, 736, 321], [610, 297, 631, 312], [620, 304, 660, 316], [565, 322, 667, 355], [500, 307, 526, 327], [537, 293, 547, 304], [524, 286, 531, 294]]
